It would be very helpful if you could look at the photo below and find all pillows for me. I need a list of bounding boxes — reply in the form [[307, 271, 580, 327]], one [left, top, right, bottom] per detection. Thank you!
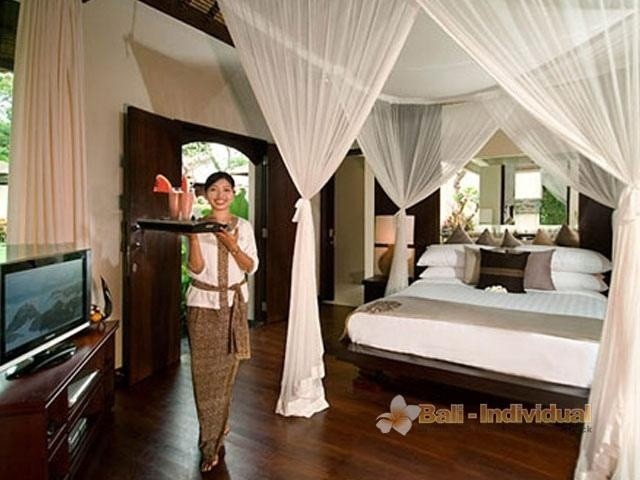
[[417, 223, 612, 294]]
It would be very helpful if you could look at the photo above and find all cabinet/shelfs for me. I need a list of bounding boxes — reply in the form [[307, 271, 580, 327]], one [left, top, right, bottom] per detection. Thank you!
[[0, 319, 120, 479]]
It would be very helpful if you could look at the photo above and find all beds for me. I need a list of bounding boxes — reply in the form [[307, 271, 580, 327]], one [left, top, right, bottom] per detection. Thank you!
[[345, 280, 609, 426]]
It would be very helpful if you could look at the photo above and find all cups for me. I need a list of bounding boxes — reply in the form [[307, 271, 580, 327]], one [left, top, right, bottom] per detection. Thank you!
[[181, 193, 194, 222], [168, 191, 184, 221]]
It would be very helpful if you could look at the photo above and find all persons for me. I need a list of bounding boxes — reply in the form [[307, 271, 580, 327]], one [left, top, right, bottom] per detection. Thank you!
[[182, 171, 261, 473]]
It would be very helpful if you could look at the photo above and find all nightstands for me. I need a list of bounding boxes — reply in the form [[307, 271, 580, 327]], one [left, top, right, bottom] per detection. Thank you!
[[362, 275, 419, 303]]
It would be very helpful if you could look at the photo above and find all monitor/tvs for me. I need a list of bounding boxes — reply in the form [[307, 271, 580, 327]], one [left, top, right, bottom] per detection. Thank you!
[[0, 247, 92, 377]]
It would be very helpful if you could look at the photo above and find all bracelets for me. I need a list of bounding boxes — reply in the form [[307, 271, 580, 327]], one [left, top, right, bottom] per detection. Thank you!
[[229, 247, 240, 257]]
